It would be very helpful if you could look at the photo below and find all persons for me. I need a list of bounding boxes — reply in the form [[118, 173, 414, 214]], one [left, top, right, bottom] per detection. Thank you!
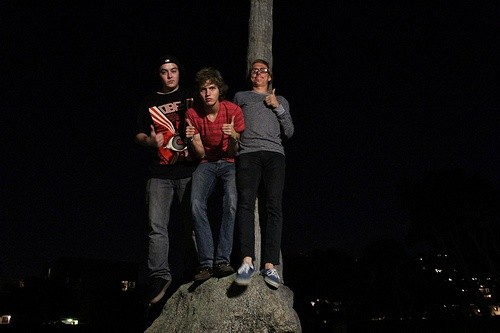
[[131, 50, 200, 304], [184, 66, 246, 281], [232, 59, 294, 290]]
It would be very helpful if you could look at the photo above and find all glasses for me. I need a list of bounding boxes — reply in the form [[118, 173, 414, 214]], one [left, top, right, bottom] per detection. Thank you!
[[250, 68, 269, 75]]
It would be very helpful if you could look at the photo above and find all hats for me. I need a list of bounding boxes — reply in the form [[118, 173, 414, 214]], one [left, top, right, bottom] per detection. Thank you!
[[157, 54, 181, 71]]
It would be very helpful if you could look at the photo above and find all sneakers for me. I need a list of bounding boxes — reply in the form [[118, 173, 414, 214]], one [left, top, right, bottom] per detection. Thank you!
[[194, 267, 213, 280], [150, 277, 171, 303], [235, 263, 255, 286], [213, 263, 235, 279], [262, 269, 281, 288]]
